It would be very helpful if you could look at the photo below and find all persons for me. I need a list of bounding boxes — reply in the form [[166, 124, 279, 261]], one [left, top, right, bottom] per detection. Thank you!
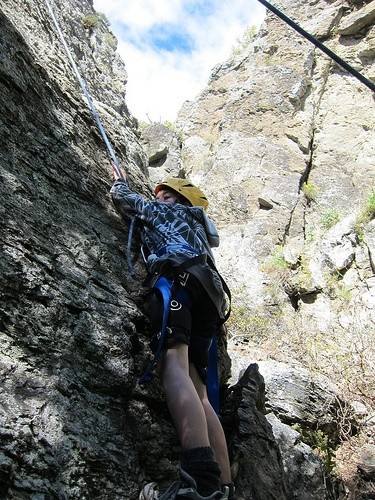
[[111, 165, 237, 500]]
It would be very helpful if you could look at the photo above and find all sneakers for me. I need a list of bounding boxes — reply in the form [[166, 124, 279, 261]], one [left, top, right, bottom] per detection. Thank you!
[[137, 466, 230, 500]]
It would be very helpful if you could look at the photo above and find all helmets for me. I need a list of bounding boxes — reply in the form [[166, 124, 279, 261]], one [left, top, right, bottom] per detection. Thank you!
[[155, 178, 208, 212]]
